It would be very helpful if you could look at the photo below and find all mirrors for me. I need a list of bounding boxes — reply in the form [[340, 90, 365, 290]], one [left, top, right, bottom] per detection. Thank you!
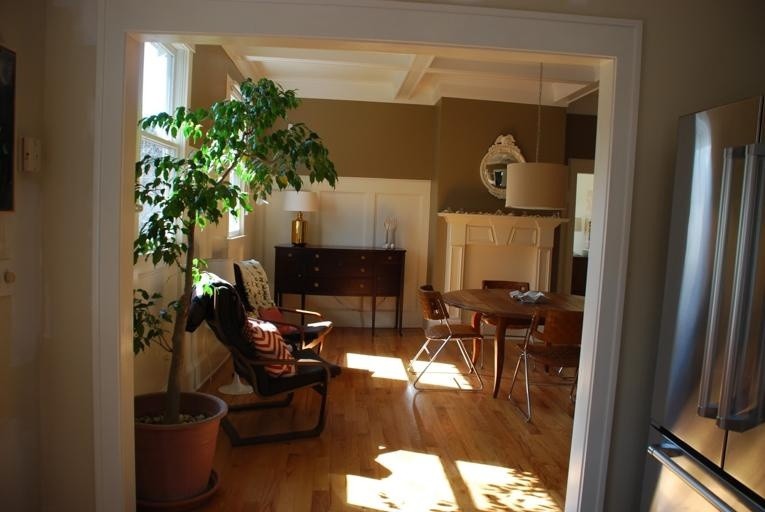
[[479, 133, 526, 201]]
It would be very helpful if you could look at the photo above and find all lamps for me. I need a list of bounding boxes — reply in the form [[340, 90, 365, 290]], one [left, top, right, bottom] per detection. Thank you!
[[506, 62, 570, 211], [282, 190, 318, 245]]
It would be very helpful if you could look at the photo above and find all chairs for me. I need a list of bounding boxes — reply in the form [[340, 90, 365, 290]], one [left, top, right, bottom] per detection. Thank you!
[[508, 309, 584, 423], [407, 284, 485, 392], [481, 280, 536, 369], [233, 260, 333, 353], [186, 272, 342, 447]]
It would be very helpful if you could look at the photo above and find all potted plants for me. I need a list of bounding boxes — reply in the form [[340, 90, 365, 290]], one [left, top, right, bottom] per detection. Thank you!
[[132, 76, 340, 512]]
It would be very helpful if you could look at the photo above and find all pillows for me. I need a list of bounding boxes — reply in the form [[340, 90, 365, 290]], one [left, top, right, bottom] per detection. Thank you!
[[247, 317, 297, 378], [258, 304, 297, 335]]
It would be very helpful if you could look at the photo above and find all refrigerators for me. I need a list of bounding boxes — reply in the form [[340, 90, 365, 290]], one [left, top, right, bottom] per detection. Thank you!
[[639, 95, 765, 512]]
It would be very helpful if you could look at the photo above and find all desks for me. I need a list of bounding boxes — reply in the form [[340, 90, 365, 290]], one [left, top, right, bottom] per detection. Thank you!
[[273, 244, 407, 337], [441, 290, 585, 398]]
